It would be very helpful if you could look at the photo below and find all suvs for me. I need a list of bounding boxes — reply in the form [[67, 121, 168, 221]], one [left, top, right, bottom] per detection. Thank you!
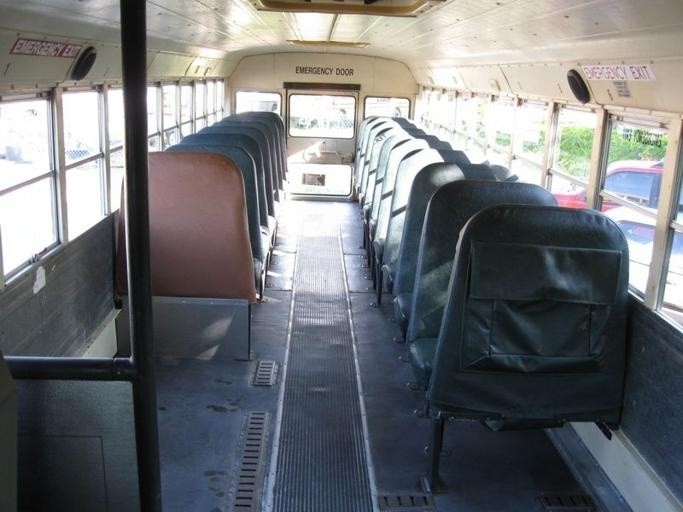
[[540, 156, 682, 326]]
